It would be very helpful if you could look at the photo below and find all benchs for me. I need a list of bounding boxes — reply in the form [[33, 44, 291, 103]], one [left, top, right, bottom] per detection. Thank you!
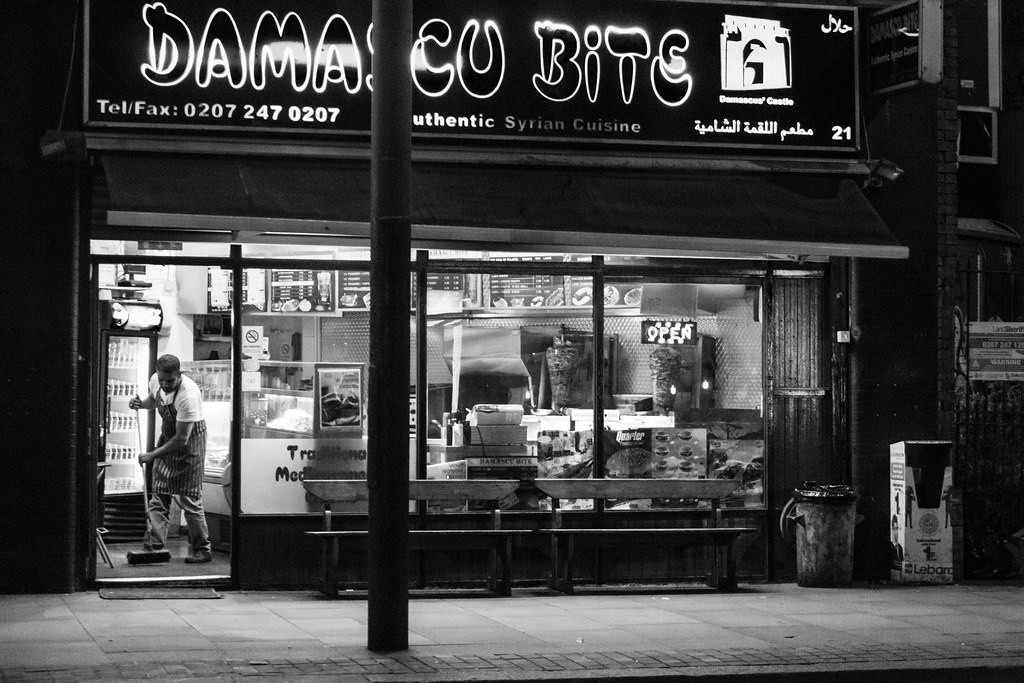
[[301, 479, 532, 596], [534, 478, 758, 591]]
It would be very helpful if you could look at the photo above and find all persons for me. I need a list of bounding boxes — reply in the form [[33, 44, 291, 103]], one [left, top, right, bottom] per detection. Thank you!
[[127, 355, 212, 563]]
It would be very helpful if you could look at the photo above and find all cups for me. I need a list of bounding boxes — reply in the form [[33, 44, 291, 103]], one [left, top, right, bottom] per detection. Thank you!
[[317, 272, 332, 304]]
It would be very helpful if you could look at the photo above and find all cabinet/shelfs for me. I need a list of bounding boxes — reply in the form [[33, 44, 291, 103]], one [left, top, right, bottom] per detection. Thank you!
[[243, 361, 364, 437]]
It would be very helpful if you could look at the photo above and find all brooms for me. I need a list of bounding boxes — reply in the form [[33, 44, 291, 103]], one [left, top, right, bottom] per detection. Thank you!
[[126, 396, 172, 565]]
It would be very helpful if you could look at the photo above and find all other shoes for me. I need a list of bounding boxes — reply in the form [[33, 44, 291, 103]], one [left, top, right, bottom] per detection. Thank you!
[[185, 551, 212, 563], [127, 548, 146, 558]]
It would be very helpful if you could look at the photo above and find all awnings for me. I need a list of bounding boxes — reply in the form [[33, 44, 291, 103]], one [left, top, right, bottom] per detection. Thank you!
[[85, 136, 910, 266]]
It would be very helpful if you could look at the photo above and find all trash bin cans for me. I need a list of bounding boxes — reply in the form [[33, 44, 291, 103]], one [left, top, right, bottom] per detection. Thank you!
[[786, 481, 859, 586]]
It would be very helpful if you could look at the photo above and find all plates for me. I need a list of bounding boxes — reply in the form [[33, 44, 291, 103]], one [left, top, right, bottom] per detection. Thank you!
[[602, 285, 620, 308], [624, 287, 642, 306], [572, 286, 592, 307]]
